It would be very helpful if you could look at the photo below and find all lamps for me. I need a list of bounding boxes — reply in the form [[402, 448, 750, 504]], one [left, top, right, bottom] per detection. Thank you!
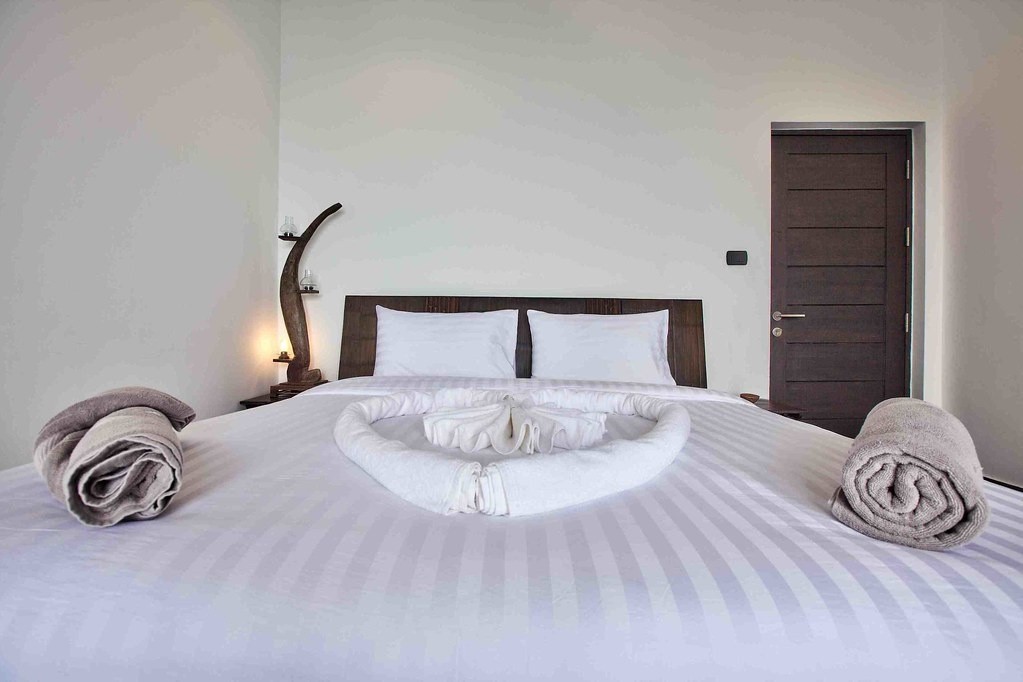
[[270, 203, 342, 397]]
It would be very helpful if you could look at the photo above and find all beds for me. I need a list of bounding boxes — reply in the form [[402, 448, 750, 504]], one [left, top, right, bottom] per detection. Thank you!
[[1, 295, 1022, 682]]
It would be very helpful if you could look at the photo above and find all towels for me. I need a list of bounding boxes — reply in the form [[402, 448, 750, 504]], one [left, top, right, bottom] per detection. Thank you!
[[332, 388, 692, 514], [35, 386, 196, 526], [827, 396, 993, 552]]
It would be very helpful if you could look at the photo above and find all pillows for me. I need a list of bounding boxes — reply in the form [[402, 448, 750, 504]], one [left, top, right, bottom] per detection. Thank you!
[[374, 304, 518, 378], [526, 309, 676, 385]]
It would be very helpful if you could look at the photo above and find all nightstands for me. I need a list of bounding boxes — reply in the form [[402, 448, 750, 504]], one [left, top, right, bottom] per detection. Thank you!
[[754, 399, 806, 420], [241, 394, 280, 408]]
